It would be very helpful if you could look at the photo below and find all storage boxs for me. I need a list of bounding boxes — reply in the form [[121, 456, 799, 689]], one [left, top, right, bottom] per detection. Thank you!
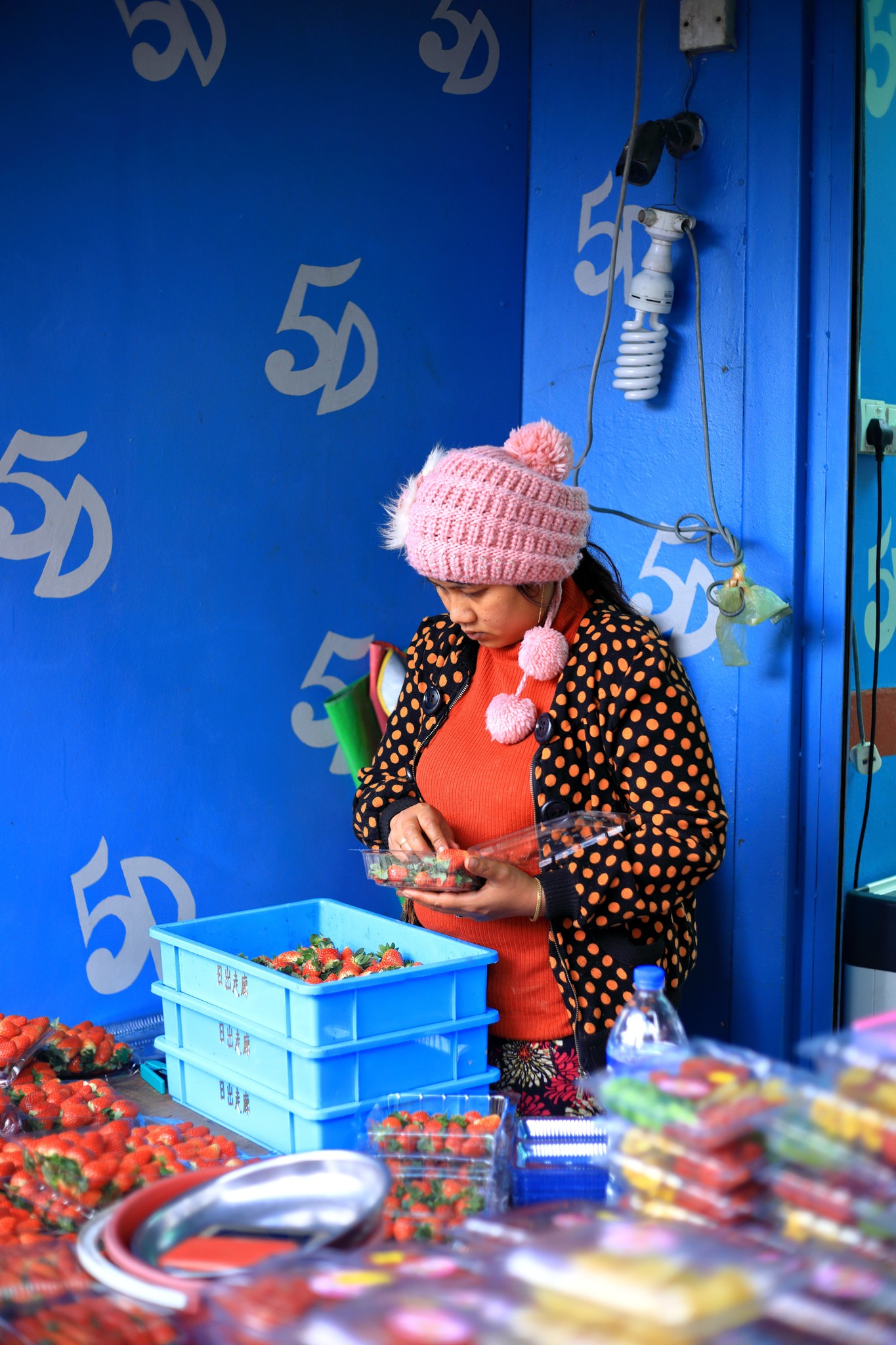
[[0, 897, 896, 1345]]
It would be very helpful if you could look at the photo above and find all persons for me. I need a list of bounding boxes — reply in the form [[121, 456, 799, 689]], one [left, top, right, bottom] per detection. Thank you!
[[349, 422, 726, 1121]]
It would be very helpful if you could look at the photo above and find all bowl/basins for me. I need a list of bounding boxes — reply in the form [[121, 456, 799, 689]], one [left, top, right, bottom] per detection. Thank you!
[[1, 1006, 896, 1345], [362, 805, 625, 891]]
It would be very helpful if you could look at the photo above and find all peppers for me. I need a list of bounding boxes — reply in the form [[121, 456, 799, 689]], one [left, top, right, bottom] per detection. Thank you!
[[597, 1051, 896, 1261]]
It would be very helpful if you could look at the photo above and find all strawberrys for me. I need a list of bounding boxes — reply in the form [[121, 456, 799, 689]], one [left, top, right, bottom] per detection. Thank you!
[[370, 1109, 502, 1244], [0, 1119, 260, 1345], [367, 850, 510, 892], [0, 1014, 134, 1147], [246, 931, 425, 985]]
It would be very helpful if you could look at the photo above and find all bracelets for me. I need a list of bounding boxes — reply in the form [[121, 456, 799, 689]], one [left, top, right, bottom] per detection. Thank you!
[[529, 878, 541, 922]]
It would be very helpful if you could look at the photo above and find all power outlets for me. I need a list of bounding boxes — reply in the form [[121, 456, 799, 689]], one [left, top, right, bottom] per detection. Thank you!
[[862, 399, 892, 458]]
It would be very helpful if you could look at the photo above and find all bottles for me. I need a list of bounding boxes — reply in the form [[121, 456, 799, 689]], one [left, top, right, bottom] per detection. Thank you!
[[604, 965, 694, 1207]]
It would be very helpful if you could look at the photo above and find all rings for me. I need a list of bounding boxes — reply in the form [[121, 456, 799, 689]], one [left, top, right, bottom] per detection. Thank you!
[[399, 838, 407, 848]]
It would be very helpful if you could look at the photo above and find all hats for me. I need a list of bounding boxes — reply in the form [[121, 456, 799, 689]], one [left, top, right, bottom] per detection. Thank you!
[[380, 419, 593, 746]]
